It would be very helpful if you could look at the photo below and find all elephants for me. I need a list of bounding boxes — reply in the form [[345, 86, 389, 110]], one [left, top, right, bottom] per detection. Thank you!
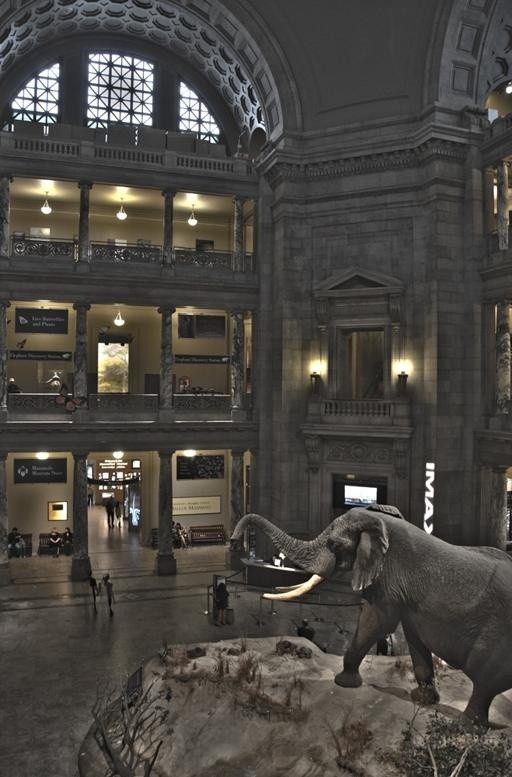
[[230, 504, 512, 728]]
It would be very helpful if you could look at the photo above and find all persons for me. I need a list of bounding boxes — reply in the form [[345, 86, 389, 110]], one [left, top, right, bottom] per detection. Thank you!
[[59, 380, 68, 394], [105, 493, 121, 528], [191, 386, 203, 394], [297, 618, 315, 641], [171, 521, 186, 549], [86, 483, 93, 506], [6, 527, 21, 560], [88, 570, 115, 616], [50, 527, 73, 559], [7, 381, 20, 393], [215, 582, 230, 626], [376, 633, 392, 656]]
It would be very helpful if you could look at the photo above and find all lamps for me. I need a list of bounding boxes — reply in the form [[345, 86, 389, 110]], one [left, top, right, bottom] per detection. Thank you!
[[115, 197, 127, 220], [188, 204, 198, 227], [40, 191, 52, 215]]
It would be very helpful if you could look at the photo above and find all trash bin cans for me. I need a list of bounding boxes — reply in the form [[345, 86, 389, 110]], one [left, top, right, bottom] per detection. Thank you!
[[225, 609, 234, 624], [152, 528, 160, 549]]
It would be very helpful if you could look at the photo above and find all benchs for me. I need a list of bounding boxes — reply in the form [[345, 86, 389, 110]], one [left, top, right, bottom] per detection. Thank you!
[[152, 527, 189, 548], [18, 533, 33, 556], [189, 525, 227, 546], [38, 533, 73, 554]]
